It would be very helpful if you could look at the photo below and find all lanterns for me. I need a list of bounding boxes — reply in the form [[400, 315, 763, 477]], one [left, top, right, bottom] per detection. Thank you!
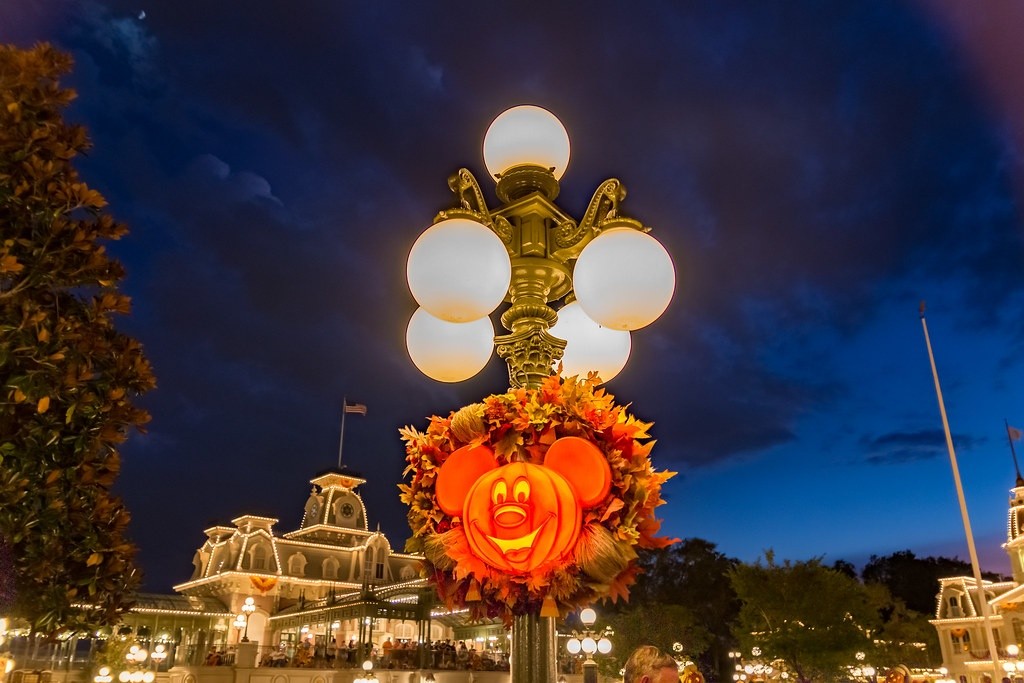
[[436, 436, 612, 572]]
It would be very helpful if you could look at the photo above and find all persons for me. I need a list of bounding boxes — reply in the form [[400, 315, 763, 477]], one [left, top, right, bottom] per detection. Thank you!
[[623, 645, 680, 683], [680, 666, 704, 683], [206, 639, 513, 673]]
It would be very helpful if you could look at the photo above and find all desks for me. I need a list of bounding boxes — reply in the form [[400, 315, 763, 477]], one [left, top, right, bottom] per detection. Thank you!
[[203, 639, 510, 669]]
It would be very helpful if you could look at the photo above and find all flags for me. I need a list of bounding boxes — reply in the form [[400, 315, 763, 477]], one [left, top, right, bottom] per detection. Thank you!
[[345, 397, 367, 415]]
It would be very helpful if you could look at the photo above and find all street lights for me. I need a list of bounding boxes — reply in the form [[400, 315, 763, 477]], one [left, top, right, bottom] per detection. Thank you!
[[151, 644, 167, 683], [566, 608, 612, 683], [405, 103, 675, 683]]
